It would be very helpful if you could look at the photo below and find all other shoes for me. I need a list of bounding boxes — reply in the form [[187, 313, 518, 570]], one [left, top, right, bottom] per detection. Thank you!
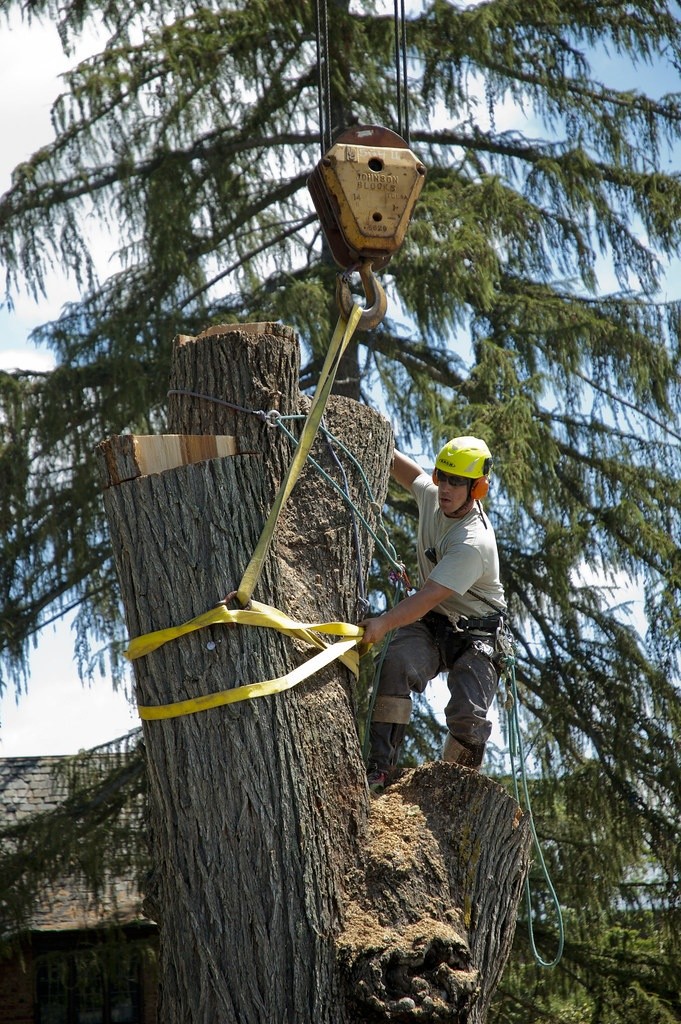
[[367, 768, 390, 793]]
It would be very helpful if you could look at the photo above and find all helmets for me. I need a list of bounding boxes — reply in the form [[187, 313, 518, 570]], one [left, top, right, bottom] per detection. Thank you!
[[435, 435, 492, 479]]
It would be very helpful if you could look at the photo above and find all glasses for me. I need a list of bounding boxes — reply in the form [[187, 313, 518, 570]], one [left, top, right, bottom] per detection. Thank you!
[[437, 470, 475, 486]]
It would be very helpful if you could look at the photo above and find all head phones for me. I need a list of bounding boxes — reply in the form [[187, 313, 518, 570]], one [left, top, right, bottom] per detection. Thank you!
[[432, 468, 489, 499]]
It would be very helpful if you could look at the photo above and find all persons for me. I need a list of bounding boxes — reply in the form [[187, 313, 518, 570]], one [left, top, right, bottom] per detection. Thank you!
[[357, 436, 507, 794]]
